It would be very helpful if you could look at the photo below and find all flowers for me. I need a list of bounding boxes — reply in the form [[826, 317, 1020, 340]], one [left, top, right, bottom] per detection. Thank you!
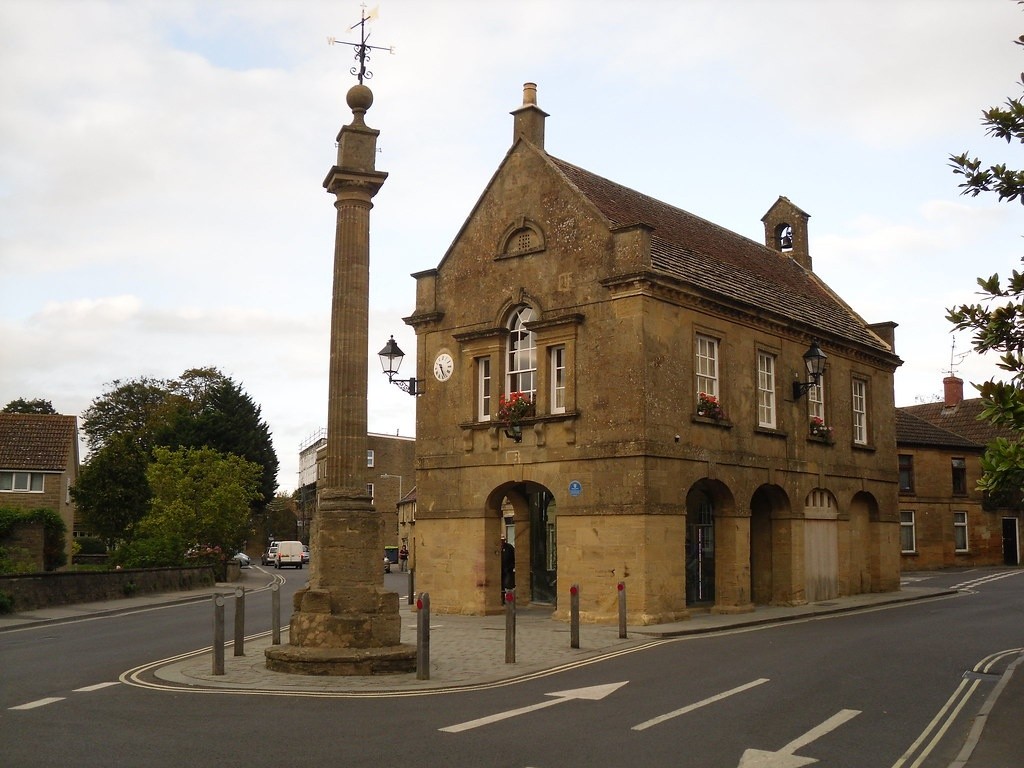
[[810, 416, 834, 437], [495, 391, 531, 425], [697, 393, 727, 421]]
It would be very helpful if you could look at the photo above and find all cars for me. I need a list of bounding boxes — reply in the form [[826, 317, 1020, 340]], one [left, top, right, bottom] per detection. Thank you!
[[302, 544, 310, 564], [232, 552, 250, 568], [383, 549, 390, 573], [269, 541, 280, 547]]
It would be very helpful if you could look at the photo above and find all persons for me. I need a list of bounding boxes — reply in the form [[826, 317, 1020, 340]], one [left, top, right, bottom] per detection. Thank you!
[[501, 534, 516, 603], [399, 545, 409, 572]]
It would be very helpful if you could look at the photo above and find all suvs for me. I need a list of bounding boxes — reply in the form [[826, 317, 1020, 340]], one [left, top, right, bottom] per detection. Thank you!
[[260, 546, 277, 565]]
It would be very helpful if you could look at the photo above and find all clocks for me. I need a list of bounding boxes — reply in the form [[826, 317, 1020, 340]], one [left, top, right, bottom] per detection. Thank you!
[[433, 354, 454, 382]]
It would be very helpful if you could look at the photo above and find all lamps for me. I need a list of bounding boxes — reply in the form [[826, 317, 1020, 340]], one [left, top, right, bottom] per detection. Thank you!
[[377, 335, 425, 396], [793, 337, 826, 401]]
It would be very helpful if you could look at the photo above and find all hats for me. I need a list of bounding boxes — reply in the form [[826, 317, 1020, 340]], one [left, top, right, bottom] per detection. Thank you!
[[501, 534, 507, 540]]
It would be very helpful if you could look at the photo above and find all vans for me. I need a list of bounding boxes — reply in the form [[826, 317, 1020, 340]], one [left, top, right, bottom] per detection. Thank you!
[[273, 540, 304, 569]]
